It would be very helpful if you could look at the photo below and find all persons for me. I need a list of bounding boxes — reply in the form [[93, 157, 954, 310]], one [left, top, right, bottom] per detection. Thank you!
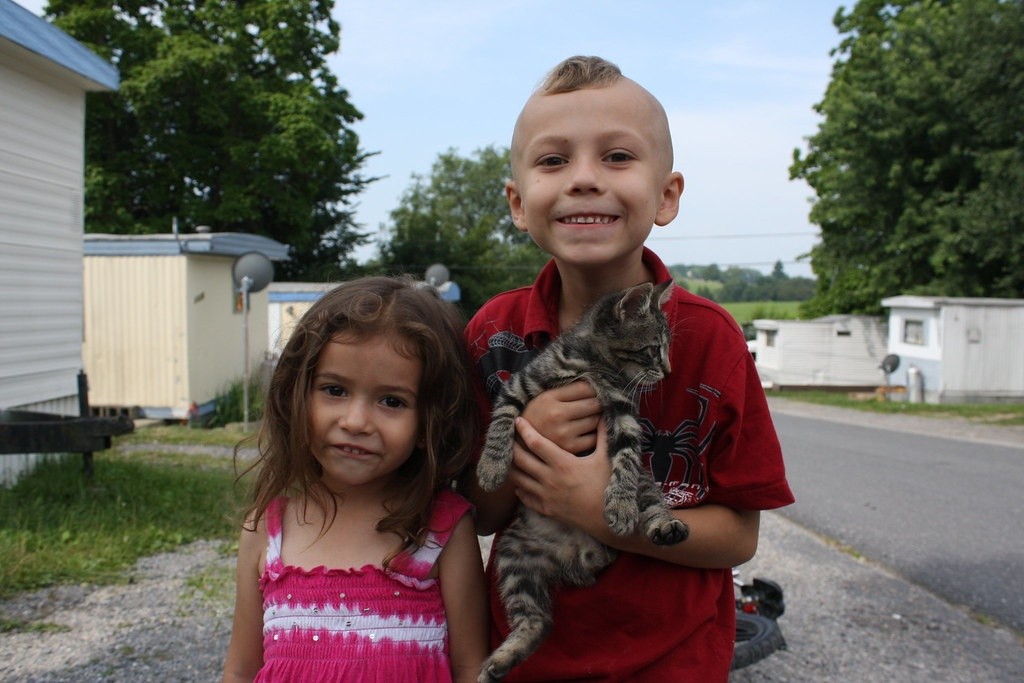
[[462, 56, 796, 682], [219, 276, 496, 683]]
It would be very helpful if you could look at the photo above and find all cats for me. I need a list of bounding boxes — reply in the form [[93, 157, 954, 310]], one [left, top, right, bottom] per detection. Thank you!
[[471, 276, 691, 683]]
